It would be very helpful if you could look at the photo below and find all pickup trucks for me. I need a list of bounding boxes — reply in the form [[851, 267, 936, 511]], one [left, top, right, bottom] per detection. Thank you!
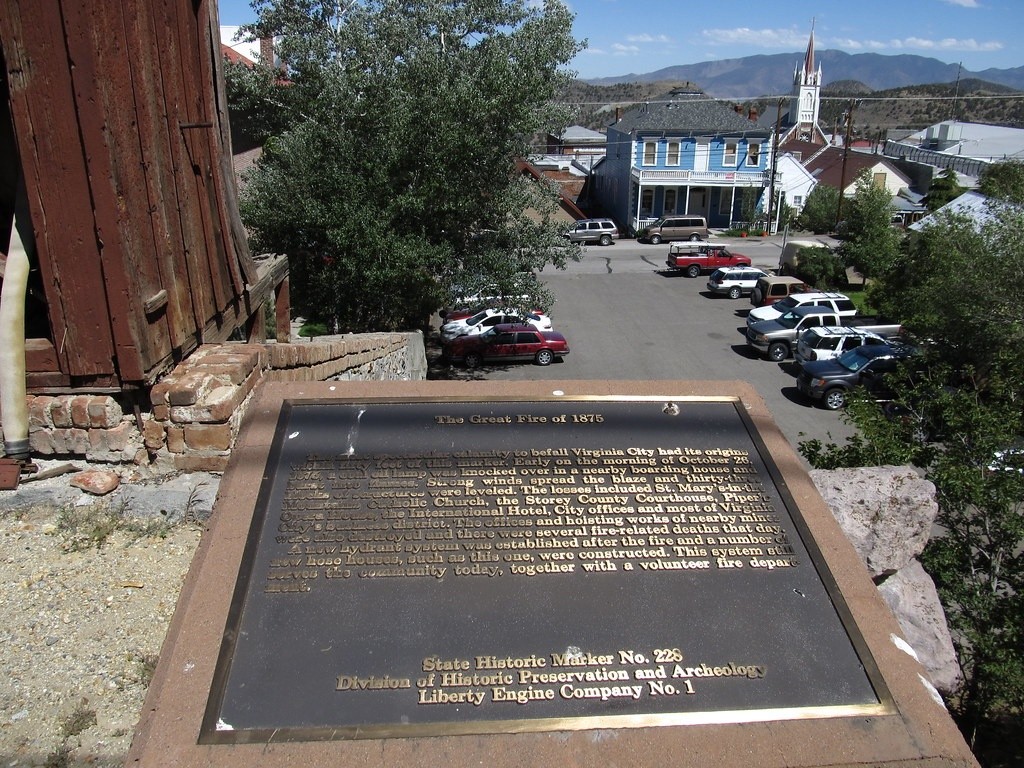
[[746, 304, 906, 362], [665, 242, 753, 278]]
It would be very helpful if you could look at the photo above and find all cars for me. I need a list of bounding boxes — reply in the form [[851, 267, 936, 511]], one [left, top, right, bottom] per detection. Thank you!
[[442, 323, 570, 370], [439, 307, 554, 346], [439, 276, 549, 324]]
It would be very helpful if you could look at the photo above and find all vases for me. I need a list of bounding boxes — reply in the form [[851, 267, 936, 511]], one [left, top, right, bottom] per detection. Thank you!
[[741, 232, 746, 237], [761, 231, 766, 236]]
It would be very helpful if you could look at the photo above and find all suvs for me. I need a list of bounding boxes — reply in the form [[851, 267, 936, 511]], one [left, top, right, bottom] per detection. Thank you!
[[641, 213, 713, 245], [707, 265, 770, 299], [796, 344, 926, 412], [750, 276, 821, 310], [746, 292, 858, 328], [561, 217, 620, 246], [792, 324, 905, 370]]
